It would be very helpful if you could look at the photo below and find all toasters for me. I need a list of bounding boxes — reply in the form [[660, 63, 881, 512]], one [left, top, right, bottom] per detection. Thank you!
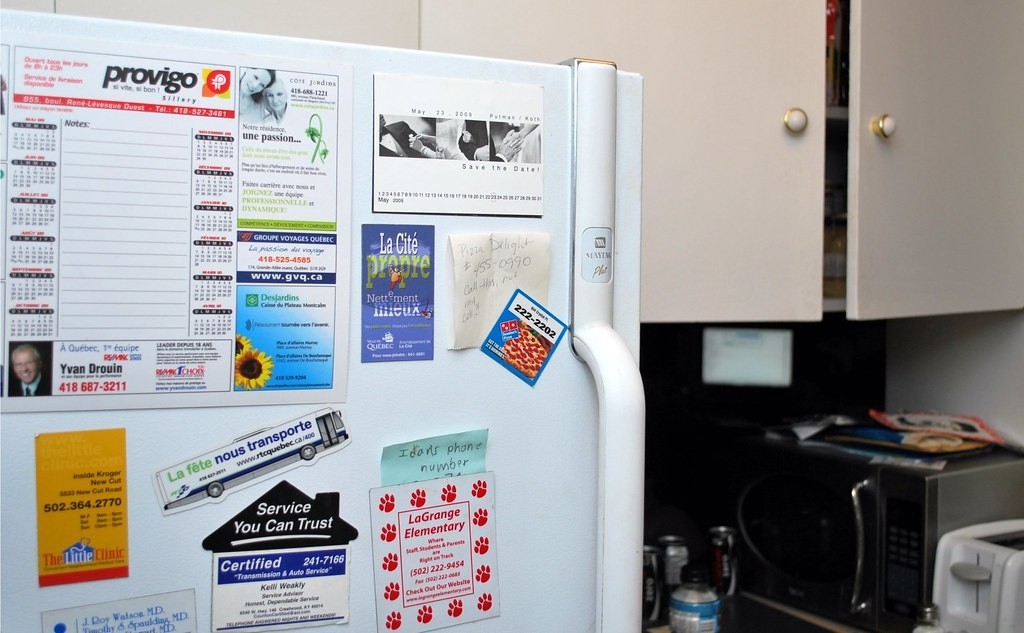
[[931, 518, 1024, 633]]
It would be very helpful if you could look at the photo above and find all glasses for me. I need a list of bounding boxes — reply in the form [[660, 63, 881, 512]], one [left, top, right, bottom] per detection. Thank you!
[[14, 359, 39, 366]]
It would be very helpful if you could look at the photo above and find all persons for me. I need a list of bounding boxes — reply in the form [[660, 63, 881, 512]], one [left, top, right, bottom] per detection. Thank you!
[[9, 344, 52, 397], [239, 68, 288, 124], [377, 113, 540, 162]]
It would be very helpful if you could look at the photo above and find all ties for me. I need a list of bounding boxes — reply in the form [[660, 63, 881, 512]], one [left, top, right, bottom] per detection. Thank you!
[[25, 386, 31, 396]]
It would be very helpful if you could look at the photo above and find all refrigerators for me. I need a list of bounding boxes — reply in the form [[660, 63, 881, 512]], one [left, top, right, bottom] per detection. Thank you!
[[0, 6, 646, 633]]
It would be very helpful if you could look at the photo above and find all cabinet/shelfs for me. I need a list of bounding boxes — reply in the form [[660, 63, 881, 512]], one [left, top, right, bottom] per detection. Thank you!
[[419, 1, 1024, 320]]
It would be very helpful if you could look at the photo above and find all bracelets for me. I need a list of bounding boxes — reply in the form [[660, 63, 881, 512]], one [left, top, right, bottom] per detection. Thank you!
[[421, 147, 427, 155]]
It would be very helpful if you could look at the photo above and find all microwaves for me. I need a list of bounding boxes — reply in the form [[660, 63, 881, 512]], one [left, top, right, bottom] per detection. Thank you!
[[730, 428, 1024, 633]]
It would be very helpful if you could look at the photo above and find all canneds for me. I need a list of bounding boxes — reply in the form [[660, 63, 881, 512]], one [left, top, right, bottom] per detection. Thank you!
[[642, 536, 691, 628], [708, 524, 739, 597]]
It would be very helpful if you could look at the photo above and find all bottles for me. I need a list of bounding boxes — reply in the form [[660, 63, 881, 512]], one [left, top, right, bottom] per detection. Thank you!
[[910, 605, 946, 633], [668, 565, 723, 633]]
